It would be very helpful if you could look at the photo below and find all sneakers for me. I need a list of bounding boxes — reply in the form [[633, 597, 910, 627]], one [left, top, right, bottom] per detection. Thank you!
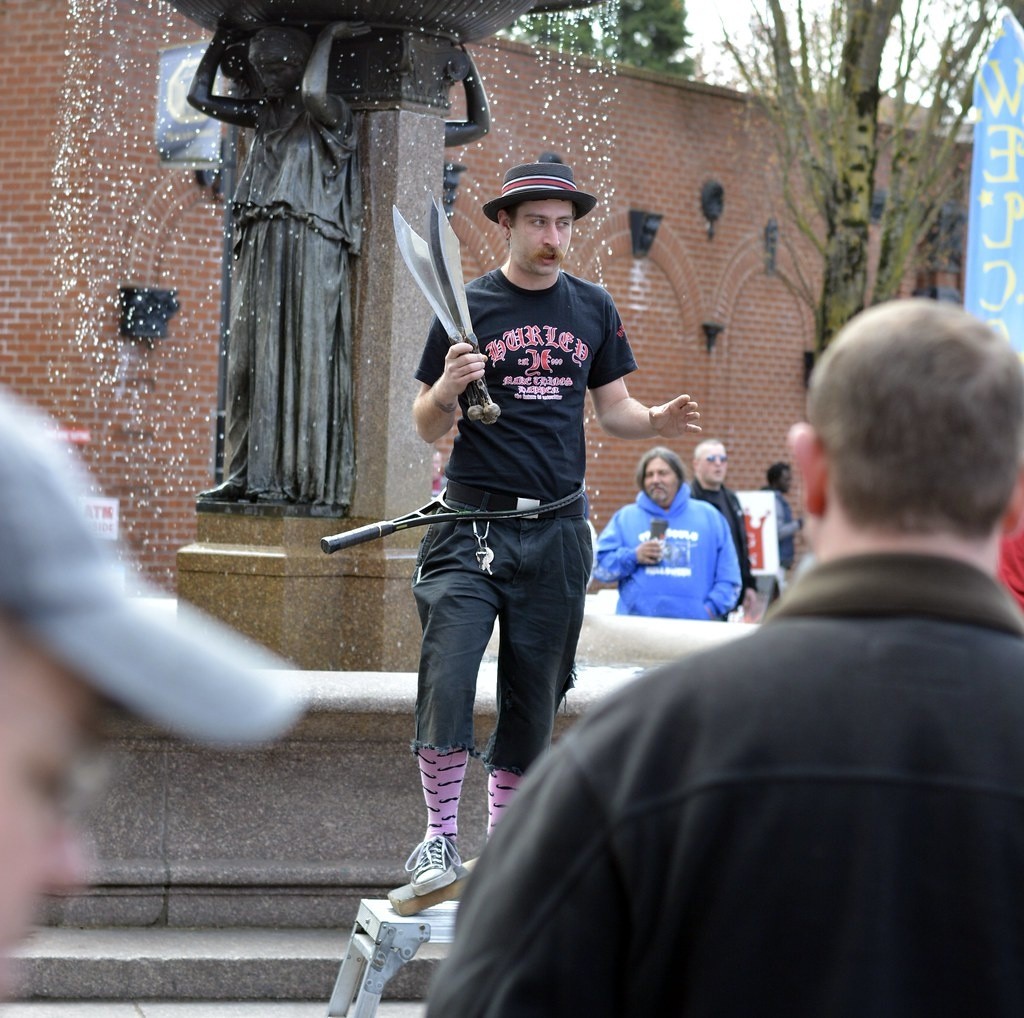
[[405, 835, 464, 897]]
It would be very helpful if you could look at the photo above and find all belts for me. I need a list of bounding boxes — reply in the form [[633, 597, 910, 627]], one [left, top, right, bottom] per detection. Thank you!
[[445, 481, 584, 522]]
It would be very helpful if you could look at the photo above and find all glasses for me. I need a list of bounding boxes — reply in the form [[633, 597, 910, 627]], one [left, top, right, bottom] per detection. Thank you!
[[699, 454, 729, 463]]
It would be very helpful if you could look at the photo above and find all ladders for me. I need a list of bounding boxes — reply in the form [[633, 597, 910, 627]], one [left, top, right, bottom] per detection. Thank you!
[[327, 900, 459, 1018]]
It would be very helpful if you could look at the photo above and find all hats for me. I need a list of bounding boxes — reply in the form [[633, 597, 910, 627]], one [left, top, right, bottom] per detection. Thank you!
[[480, 163, 597, 221], [0, 390, 311, 749]]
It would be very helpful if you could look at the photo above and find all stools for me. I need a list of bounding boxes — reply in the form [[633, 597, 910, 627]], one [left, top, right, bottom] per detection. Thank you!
[[326, 898, 459, 1018]]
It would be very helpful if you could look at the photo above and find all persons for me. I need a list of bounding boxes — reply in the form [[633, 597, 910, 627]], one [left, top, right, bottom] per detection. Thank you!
[[187, 12, 372, 505], [594, 446, 742, 620], [1, 390, 310, 950], [425, 297, 1023, 1017], [444, 43, 493, 216], [689, 440, 757, 613], [404, 162, 703, 897], [762, 463, 803, 575]]
